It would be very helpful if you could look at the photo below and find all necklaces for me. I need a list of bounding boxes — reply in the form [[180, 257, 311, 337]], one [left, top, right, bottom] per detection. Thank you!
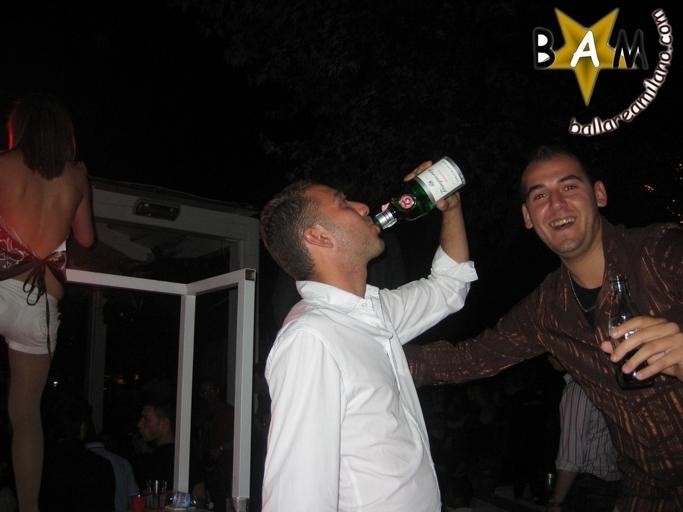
[[563, 268, 601, 314]]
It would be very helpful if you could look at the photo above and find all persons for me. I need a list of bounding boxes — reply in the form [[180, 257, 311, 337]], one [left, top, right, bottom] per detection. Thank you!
[[39, 372, 233, 511], [0, 93, 95, 511], [400, 143, 683, 511], [545, 353, 623, 511], [258, 160, 479, 512]]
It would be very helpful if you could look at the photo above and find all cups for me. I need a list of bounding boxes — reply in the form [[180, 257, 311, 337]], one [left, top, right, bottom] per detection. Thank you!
[[129, 480, 168, 511]]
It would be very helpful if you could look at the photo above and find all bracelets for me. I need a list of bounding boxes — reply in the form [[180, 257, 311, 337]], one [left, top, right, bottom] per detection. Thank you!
[[549, 500, 566, 508]]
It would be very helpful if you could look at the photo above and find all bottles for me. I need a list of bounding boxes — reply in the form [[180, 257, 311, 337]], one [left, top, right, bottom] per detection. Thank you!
[[607, 275, 656, 388], [371, 154, 466, 229], [166, 492, 200, 507]]
